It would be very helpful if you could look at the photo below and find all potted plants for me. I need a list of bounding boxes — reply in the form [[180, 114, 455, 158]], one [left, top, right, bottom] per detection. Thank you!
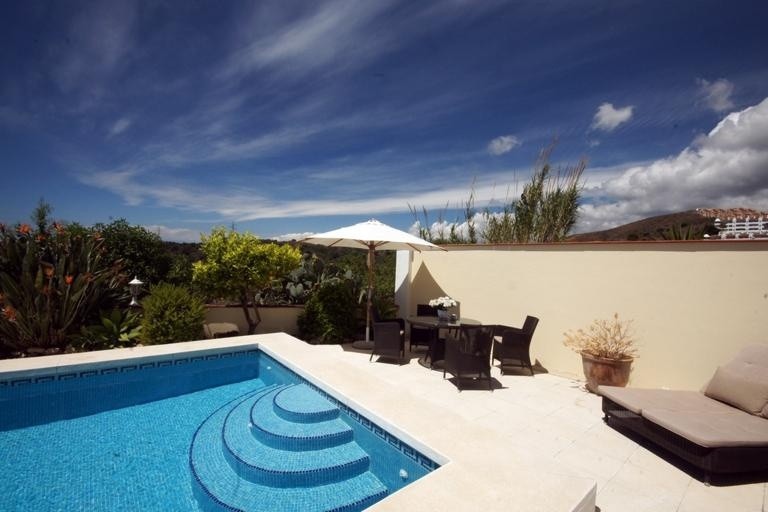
[[564, 311, 639, 394]]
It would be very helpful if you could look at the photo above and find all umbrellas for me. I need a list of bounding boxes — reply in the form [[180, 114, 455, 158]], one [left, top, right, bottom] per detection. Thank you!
[[296, 218, 450, 342]]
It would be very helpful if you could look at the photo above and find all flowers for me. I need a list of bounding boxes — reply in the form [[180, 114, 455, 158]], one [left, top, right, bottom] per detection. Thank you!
[[429, 296, 458, 311]]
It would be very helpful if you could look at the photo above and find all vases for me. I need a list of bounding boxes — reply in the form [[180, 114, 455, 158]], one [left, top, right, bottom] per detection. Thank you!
[[437, 308, 449, 322]]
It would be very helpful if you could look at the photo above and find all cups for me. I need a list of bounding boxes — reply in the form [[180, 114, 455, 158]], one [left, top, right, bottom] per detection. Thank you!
[[450, 314, 456, 322]]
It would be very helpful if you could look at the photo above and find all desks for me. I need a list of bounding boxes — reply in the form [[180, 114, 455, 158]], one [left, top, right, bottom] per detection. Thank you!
[[407, 315, 482, 370]]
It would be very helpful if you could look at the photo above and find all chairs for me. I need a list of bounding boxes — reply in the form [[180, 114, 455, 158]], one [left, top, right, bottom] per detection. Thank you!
[[597, 343, 768, 488], [409, 304, 442, 352], [491, 315, 539, 376], [369, 322, 405, 366], [444, 325, 496, 391]]
